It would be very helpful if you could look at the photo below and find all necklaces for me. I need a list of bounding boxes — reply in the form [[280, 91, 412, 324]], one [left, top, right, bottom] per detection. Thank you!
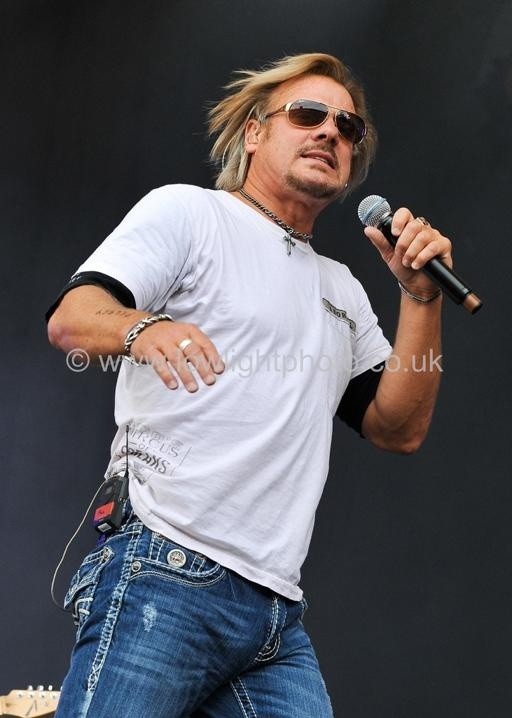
[[238, 187, 313, 256]]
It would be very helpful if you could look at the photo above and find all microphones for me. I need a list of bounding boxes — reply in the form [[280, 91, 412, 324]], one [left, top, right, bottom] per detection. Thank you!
[[357, 194, 482, 315]]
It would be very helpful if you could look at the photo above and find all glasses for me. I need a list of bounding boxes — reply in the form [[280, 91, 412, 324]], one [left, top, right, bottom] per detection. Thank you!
[[256, 97, 369, 146]]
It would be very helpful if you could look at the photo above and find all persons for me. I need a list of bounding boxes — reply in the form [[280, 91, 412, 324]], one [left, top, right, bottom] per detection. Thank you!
[[46, 55, 484, 717]]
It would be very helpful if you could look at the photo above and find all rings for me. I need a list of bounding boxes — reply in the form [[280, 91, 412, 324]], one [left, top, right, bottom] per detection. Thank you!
[[178, 338, 192, 351], [416, 216, 428, 225]]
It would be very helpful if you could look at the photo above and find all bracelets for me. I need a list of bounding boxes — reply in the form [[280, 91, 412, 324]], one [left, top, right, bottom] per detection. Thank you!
[[124, 313, 173, 355], [396, 280, 441, 304]]
